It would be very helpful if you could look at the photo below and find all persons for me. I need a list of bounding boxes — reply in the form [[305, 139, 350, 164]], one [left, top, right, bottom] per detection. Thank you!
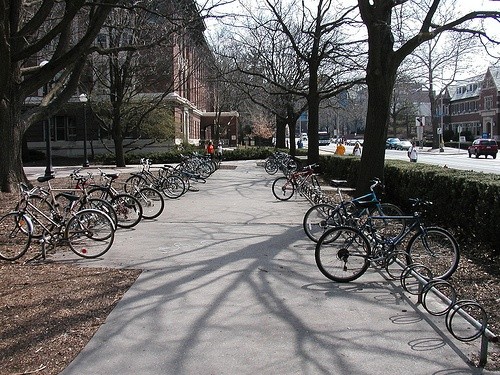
[[217, 144, 223, 161], [207, 142, 214, 154], [408, 141, 418, 162], [297, 140, 303, 148], [353, 142, 362, 158]]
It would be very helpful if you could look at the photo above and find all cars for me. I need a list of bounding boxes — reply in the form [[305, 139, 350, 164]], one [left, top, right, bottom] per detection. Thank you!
[[386, 138, 412, 150], [468, 139, 498, 159]]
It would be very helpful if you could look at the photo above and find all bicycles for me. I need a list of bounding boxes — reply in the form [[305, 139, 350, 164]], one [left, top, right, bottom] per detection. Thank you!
[[0, 167, 164, 261], [124, 154, 217, 199], [303, 178, 406, 244], [271, 163, 320, 201], [314, 197, 460, 283], [264, 150, 298, 174]]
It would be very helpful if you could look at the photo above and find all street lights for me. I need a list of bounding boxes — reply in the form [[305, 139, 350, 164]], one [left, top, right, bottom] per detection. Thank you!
[[39, 59, 56, 176], [79, 93, 90, 167]]
